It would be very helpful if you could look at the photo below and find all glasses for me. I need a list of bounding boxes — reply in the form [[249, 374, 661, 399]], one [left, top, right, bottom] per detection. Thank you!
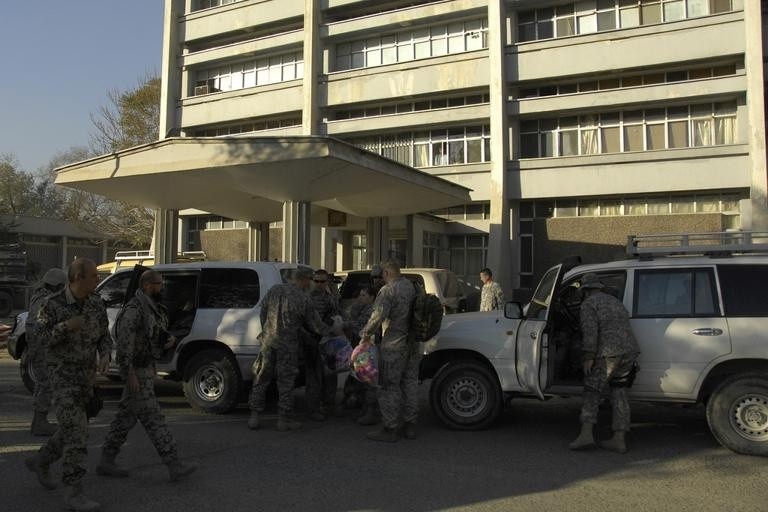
[[313, 278, 328, 283]]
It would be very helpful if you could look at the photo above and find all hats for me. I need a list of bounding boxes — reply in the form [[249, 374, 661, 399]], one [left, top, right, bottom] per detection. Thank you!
[[577, 272, 606, 292], [297, 263, 315, 274]]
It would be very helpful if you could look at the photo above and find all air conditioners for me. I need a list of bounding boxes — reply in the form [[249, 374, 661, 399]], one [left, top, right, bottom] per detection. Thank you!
[[195, 85, 208, 95]]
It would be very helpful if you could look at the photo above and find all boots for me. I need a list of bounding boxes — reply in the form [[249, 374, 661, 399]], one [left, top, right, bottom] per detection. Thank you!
[[24, 449, 58, 489], [31, 411, 58, 436], [599, 431, 628, 453], [247, 410, 259, 429], [308, 392, 416, 442], [569, 424, 595, 449], [95, 450, 129, 478], [277, 414, 302, 432], [62, 486, 100, 511]]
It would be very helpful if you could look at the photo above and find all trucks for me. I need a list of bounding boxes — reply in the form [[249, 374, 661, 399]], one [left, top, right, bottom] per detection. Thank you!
[[0, 223, 48, 319]]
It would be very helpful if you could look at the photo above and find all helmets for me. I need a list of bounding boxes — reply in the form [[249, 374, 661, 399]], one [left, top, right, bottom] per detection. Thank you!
[[41, 268, 67, 287]]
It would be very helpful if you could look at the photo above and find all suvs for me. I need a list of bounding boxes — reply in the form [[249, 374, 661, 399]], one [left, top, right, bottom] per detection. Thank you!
[[6, 259, 332, 416], [413, 230, 766, 458], [94, 247, 206, 288], [331, 267, 468, 316]]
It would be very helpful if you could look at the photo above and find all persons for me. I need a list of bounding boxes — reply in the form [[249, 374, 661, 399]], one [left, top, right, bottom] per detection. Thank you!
[[479, 268, 505, 311], [24, 259, 112, 512], [247, 257, 422, 443], [567, 272, 639, 453], [25, 268, 68, 435], [95, 269, 199, 480]]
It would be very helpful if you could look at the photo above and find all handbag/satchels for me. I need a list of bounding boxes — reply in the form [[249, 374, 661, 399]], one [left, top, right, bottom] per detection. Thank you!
[[411, 279, 444, 340]]
